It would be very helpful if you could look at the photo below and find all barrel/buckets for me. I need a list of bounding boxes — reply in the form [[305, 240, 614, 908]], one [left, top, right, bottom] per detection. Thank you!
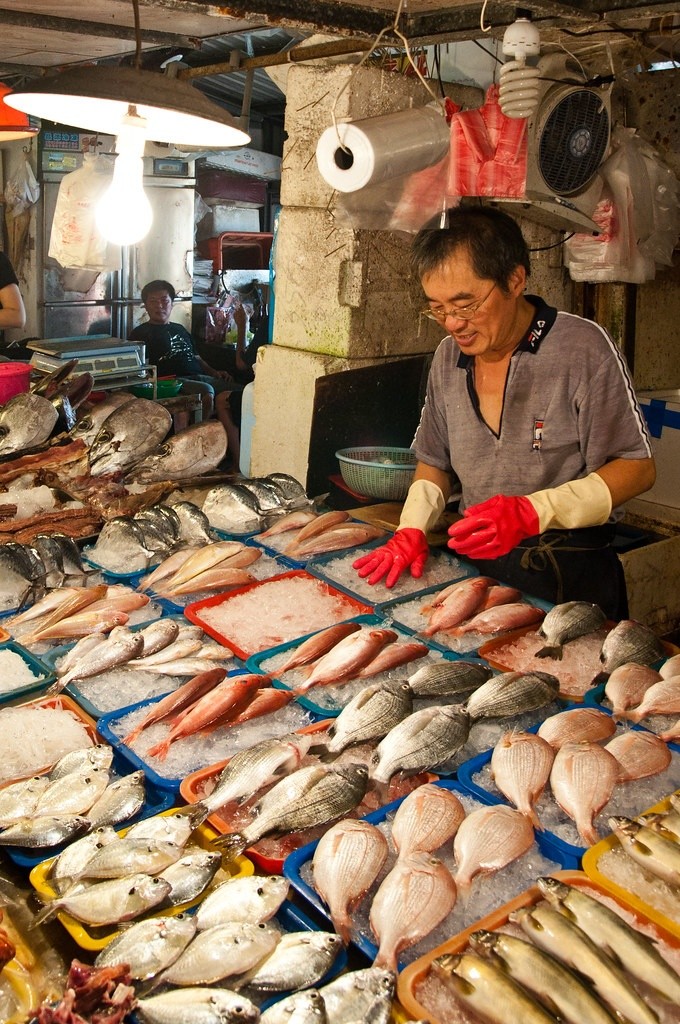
[[0, 361, 34, 408]]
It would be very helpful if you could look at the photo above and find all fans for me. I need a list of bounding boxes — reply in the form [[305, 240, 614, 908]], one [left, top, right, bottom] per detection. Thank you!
[[486, 51, 616, 236]]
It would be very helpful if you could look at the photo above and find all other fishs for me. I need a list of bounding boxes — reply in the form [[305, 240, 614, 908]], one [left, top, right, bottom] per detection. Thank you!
[[0, 359, 679, 1024]]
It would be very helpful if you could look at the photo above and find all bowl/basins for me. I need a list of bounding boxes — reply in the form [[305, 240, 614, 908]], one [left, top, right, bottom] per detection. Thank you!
[[138, 378, 183, 398]]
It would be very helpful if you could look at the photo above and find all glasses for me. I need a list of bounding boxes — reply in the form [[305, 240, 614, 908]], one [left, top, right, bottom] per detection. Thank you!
[[420, 279, 501, 321]]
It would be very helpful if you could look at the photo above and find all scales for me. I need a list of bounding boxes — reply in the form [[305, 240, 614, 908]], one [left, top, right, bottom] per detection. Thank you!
[[26, 334, 145, 382]]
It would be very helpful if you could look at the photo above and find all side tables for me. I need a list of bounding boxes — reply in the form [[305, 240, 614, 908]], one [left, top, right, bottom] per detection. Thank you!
[[195, 202, 260, 243]]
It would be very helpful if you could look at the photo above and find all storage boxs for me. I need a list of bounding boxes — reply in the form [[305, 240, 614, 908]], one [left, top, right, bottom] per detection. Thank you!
[[0, 511, 680, 1024], [193, 145, 283, 182]]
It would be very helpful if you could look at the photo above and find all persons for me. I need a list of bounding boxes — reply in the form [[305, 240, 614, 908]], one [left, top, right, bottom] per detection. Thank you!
[[129, 281, 240, 419], [215, 303, 268, 473], [352, 207, 655, 623], [0, 250, 26, 329]]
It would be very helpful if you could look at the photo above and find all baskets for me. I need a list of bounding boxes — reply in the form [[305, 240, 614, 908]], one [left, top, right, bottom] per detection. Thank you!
[[336, 445, 420, 501]]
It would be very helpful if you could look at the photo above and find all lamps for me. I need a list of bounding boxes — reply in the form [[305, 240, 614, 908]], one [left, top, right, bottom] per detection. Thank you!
[[4, 58, 252, 248], [495, 9, 544, 120]]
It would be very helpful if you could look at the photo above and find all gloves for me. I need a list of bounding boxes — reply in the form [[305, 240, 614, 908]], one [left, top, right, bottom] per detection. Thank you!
[[353, 479, 446, 589], [448, 471, 612, 559]]
